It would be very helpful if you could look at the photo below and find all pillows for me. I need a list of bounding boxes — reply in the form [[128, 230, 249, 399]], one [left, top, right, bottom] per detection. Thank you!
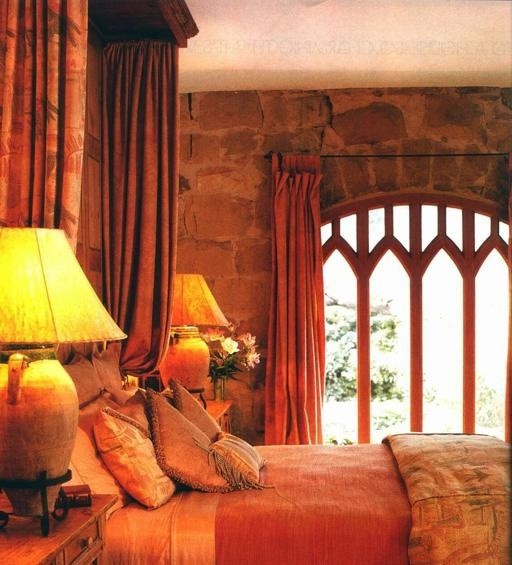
[[61, 350, 274, 520]]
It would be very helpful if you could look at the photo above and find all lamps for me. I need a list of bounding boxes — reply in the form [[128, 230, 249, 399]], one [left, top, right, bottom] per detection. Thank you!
[[0, 228, 128, 536], [159, 274, 229, 410]]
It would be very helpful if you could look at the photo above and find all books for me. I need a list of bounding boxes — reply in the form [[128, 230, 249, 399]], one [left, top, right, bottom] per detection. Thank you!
[[55, 485, 93, 509]]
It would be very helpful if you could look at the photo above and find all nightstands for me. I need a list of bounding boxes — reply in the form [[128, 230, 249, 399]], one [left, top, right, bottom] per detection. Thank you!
[[0, 493, 118, 565]]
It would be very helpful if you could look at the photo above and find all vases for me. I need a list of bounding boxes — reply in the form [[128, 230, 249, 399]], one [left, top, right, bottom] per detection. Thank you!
[[214, 378, 224, 402]]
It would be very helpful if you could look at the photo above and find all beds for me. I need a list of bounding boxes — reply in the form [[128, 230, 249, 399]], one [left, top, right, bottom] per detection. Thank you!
[[107, 445, 511, 565]]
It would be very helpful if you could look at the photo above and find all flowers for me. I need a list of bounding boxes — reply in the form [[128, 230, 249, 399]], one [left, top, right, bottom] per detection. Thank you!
[[202, 320, 260, 382]]
